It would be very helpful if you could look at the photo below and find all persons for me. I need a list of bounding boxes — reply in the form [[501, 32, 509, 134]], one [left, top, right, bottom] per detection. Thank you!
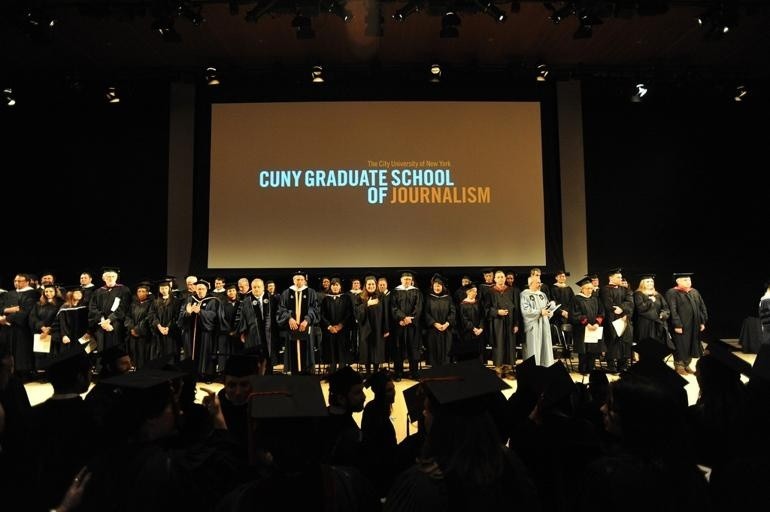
[[2, 267, 770, 511]]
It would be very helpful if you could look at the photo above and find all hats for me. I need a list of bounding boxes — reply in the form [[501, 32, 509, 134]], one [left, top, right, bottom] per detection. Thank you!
[[38, 269, 695, 294], [1, 338, 748, 413]]
